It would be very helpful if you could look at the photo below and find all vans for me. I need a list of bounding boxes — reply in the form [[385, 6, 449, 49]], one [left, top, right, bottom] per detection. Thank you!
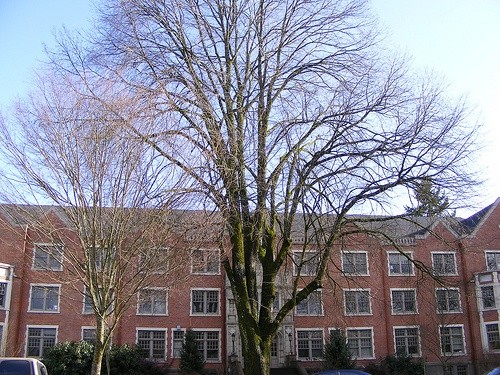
[[0, 356, 49, 375]]
